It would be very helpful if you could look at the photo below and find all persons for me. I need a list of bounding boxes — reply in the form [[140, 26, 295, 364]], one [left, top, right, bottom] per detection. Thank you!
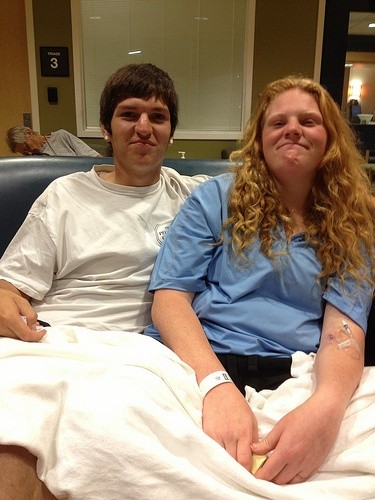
[[6, 126, 103, 157], [145, 78, 375, 485], [0, 63, 215, 500]]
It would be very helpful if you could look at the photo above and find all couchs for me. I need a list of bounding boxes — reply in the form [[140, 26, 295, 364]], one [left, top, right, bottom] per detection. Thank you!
[[0, 156, 250, 260]]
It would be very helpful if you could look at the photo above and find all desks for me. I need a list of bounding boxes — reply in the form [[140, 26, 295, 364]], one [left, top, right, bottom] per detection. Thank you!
[[347, 120, 375, 150]]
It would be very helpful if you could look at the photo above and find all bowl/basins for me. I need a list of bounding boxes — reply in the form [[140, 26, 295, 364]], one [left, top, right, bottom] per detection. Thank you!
[[357, 114, 373, 123]]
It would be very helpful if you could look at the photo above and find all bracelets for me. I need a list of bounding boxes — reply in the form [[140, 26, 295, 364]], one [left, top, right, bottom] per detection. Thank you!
[[198, 371, 233, 399]]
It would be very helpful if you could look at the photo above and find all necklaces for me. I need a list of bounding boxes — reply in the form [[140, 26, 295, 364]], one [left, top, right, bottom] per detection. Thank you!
[[43, 134, 58, 157]]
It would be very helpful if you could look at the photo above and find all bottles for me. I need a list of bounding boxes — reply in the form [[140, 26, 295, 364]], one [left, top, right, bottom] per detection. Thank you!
[[348, 100, 361, 123]]
